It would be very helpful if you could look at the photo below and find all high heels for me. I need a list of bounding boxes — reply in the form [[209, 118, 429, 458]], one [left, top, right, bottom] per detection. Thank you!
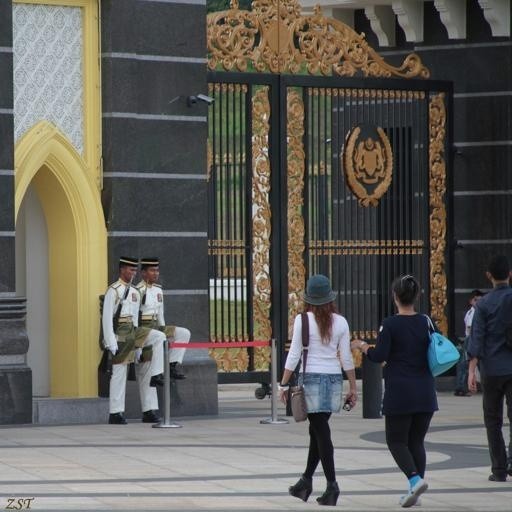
[[316, 483, 340, 506], [289, 476, 313, 501]]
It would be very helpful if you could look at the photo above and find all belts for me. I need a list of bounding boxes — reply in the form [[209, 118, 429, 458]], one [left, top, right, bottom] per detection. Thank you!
[[118, 314, 157, 323]]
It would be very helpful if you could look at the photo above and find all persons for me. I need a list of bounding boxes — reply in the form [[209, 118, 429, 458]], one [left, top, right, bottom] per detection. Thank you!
[[277, 274, 357, 506], [350, 274, 442, 507], [453, 288, 484, 397], [102, 256, 162, 425], [467, 257, 512, 481], [135, 256, 190, 423]]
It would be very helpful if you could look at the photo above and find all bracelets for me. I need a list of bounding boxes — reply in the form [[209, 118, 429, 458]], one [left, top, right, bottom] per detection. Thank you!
[[358, 341, 367, 350], [278, 384, 289, 391]]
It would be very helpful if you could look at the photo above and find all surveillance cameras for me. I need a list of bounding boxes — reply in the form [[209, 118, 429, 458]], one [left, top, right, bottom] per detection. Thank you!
[[187, 94, 197, 108], [197, 92, 215, 106]]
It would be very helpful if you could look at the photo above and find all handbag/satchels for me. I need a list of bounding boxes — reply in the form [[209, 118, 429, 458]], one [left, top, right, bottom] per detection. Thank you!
[[425, 316, 460, 377], [288, 385, 306, 422]]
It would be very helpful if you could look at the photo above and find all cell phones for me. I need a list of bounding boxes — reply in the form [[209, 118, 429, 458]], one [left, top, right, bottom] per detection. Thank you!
[[343, 396, 354, 412]]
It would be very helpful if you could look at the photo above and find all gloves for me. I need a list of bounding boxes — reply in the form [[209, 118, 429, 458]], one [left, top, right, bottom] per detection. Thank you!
[[136, 349, 142, 359], [109, 346, 119, 354]]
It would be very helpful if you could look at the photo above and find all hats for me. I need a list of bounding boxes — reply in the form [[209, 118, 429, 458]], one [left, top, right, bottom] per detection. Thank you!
[[302, 275, 336, 306], [120, 256, 140, 268], [141, 257, 160, 268]]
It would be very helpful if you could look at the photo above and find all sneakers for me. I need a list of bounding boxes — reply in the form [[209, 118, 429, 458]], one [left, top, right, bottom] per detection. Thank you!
[[489, 465, 511, 481], [398, 478, 427, 508]]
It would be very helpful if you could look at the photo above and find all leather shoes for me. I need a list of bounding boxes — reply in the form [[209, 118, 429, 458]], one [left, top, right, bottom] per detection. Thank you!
[[142, 410, 163, 423], [170, 364, 185, 380], [150, 374, 164, 387], [108, 413, 127, 424]]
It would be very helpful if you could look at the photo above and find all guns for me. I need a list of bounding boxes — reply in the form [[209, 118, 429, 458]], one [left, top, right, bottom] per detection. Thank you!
[[139, 278, 150, 327], [100, 275, 138, 370]]
